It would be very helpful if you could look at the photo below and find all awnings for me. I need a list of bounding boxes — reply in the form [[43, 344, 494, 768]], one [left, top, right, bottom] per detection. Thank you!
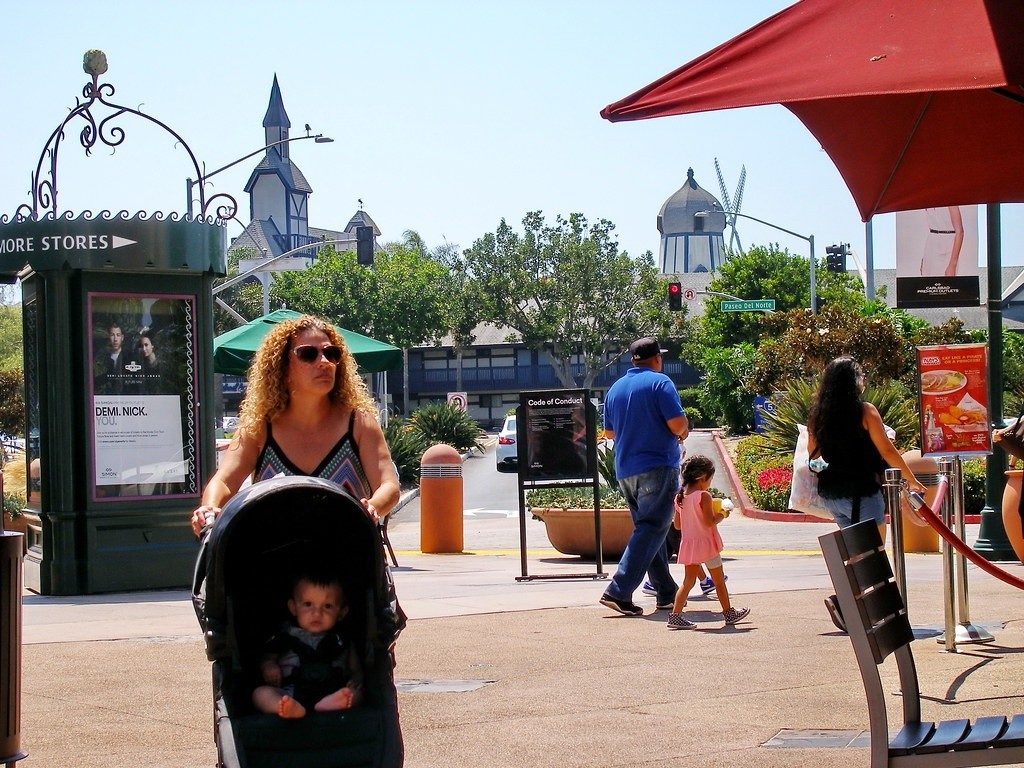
[[598, 0, 1024, 223]]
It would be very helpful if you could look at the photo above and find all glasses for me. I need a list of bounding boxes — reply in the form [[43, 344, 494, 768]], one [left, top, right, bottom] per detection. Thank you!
[[858, 372, 867, 380], [289, 345, 341, 365]]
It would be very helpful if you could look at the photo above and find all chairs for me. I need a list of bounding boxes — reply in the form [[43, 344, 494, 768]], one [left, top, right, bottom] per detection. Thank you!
[[819, 518, 1024, 768]]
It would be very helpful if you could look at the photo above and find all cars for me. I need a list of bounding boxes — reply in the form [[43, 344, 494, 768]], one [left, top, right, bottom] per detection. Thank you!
[[495, 415, 517, 472]]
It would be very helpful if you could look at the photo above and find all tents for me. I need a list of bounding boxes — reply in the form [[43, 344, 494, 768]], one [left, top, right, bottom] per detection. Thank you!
[[213, 303, 403, 427]]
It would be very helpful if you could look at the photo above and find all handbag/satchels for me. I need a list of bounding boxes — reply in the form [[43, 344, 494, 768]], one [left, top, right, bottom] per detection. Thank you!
[[788, 424, 834, 521]]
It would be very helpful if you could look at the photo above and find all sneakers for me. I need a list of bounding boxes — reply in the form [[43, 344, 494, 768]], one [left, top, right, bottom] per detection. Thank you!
[[824, 595, 847, 633], [642, 582, 658, 595], [722, 607, 750, 623], [667, 613, 697, 628], [599, 594, 643, 616], [700, 575, 728, 594], [656, 601, 687, 608]]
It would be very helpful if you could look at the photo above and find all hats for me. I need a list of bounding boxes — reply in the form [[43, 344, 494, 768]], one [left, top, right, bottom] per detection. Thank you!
[[630, 337, 668, 360]]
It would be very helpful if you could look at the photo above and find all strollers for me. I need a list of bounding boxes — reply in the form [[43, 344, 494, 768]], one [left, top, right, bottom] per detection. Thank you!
[[191, 475, 408, 768]]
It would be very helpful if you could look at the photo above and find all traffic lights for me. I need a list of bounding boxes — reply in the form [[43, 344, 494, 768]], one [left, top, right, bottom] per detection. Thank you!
[[832, 244, 846, 273], [669, 283, 681, 311], [826, 245, 838, 272]]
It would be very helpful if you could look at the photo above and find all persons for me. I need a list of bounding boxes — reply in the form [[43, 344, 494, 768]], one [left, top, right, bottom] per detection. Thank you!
[[95, 322, 172, 394], [239, 569, 364, 719], [807, 355, 928, 632], [642, 415, 728, 595], [667, 455, 751, 629], [600, 337, 689, 614], [920, 206, 964, 276], [191, 316, 400, 538]]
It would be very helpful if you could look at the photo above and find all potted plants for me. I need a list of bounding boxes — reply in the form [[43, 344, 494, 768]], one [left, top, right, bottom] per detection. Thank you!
[[2, 490, 28, 554], [527, 483, 636, 561]]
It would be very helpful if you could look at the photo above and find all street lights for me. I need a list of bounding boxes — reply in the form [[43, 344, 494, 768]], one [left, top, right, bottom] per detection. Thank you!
[[694, 210, 816, 315], [186, 134, 334, 221]]
